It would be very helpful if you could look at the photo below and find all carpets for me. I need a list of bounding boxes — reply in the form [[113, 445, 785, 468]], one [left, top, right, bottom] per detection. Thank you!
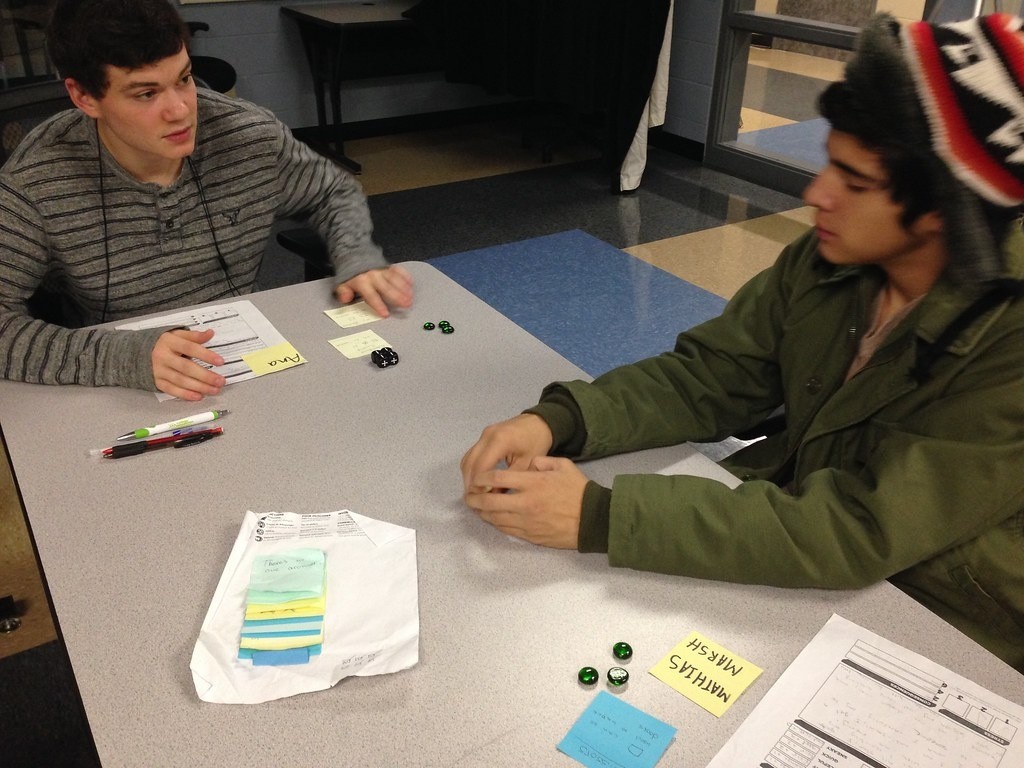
[[329, 118, 606, 197]]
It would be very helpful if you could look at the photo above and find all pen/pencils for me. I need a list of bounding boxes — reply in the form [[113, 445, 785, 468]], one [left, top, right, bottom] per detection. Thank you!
[[90, 425, 225, 459], [117, 409, 230, 442]]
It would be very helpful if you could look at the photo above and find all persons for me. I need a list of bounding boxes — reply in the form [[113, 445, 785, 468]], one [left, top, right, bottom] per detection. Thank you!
[[0, 0, 416, 400], [459, 10, 1024, 671]]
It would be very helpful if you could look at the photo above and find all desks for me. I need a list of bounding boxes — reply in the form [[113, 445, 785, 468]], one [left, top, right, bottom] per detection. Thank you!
[[0, 259, 1024, 768], [280, 2, 443, 176]]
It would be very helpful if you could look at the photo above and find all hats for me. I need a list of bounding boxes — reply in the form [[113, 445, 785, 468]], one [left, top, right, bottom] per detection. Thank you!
[[900, 12, 1024, 207]]
